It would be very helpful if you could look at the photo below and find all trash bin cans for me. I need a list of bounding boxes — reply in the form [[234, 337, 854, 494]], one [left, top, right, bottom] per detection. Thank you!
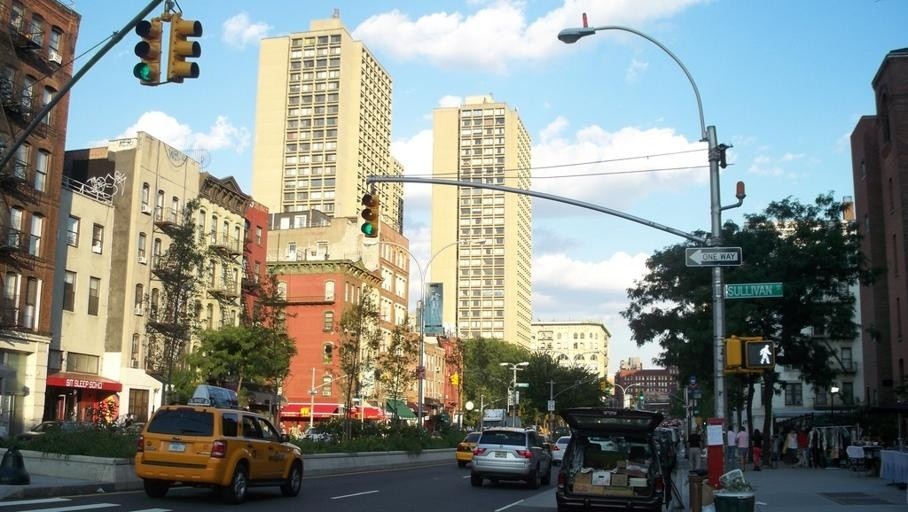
[[689, 469, 714, 505], [713, 489, 754, 512]]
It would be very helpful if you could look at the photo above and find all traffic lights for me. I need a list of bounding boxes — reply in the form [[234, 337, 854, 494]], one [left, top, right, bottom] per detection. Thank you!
[[133, 19, 164, 86], [640, 390, 644, 400], [166, 12, 202, 87], [361, 193, 379, 238], [745, 337, 775, 373], [721, 334, 743, 374]]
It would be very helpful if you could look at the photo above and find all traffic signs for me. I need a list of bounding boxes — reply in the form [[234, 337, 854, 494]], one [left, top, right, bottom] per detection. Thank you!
[[684, 244, 742, 268], [724, 283, 783, 300]]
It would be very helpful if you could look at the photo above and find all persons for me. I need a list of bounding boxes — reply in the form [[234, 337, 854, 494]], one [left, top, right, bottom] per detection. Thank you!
[[687, 428, 701, 470], [121, 413, 135, 427], [726, 425, 820, 472]]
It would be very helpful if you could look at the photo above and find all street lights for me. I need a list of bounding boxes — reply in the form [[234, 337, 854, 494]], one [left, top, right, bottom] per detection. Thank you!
[[826, 383, 840, 422], [499, 360, 529, 428], [558, 16, 739, 484], [552, 348, 611, 371], [610, 381, 640, 410]]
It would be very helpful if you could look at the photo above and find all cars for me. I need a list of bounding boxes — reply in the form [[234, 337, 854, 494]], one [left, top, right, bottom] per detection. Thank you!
[[306, 423, 334, 443], [453, 401, 684, 511], [134, 383, 307, 502], [16, 416, 147, 441], [429, 430, 441, 440]]
